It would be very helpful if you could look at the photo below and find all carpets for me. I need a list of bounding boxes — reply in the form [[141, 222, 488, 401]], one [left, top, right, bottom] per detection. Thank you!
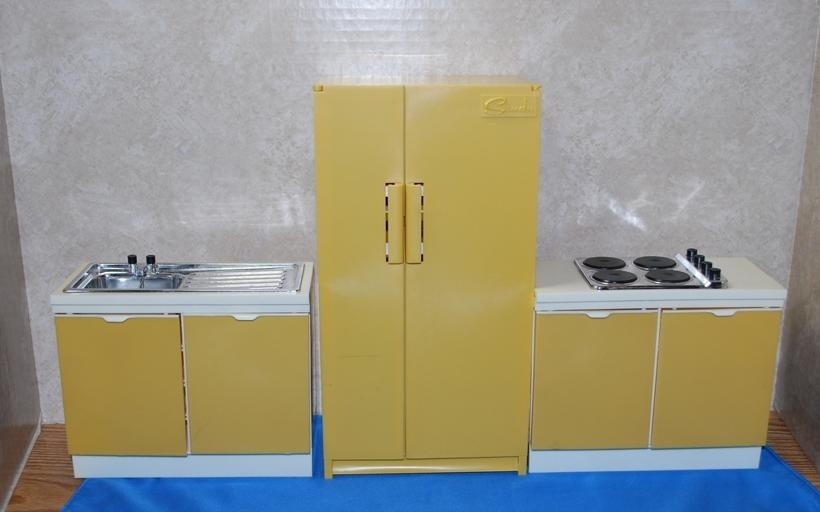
[[62, 447, 819, 512]]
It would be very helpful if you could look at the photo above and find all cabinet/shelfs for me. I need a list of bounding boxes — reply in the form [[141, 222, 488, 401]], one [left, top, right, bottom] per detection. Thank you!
[[51, 306, 312, 478], [531, 306, 783, 471]]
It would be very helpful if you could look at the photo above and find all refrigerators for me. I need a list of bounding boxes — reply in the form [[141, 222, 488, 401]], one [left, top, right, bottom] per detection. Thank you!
[[311, 82, 539, 479]]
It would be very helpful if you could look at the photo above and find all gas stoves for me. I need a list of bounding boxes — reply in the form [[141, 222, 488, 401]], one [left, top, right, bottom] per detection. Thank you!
[[573, 248, 728, 290]]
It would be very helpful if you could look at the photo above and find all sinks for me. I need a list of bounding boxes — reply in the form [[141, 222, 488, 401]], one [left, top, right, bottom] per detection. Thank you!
[[138, 263, 201, 291], [62, 261, 139, 290]]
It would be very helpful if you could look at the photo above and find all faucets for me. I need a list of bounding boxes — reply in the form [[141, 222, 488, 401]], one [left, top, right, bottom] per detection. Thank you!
[[135, 264, 148, 277]]
